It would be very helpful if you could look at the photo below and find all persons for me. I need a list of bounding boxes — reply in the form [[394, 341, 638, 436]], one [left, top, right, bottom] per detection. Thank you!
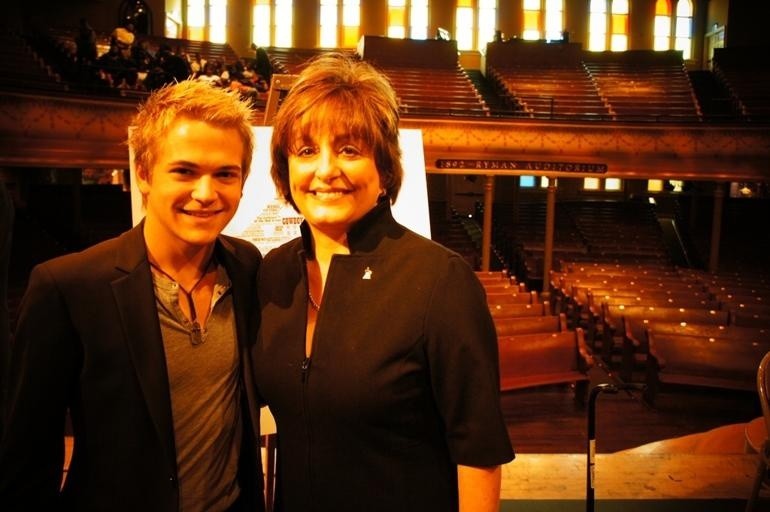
[[92, 23, 273, 85], [2, 82, 265, 511], [250, 59, 515, 511]]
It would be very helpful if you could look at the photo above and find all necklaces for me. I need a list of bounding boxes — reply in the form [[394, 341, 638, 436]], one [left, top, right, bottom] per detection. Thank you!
[[305, 279, 322, 314], [147, 255, 219, 320]]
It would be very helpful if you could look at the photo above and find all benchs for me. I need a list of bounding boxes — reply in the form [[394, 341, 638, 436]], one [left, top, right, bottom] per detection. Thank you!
[[496, 328, 594, 406], [488, 303, 554, 316], [478, 276, 518, 285], [267, 44, 358, 73], [557, 276, 704, 307], [162, 39, 242, 72], [358, 63, 489, 117], [697, 272, 770, 325], [488, 312, 571, 392], [485, 292, 537, 306], [598, 300, 722, 365], [481, 285, 526, 294], [431, 200, 507, 272], [486, 59, 702, 123], [474, 271, 508, 277], [642, 323, 767, 406], [548, 271, 696, 320], [612, 307, 730, 384], [557, 260, 692, 277], [566, 285, 709, 331], [580, 291, 713, 345], [516, 201, 670, 285]]
